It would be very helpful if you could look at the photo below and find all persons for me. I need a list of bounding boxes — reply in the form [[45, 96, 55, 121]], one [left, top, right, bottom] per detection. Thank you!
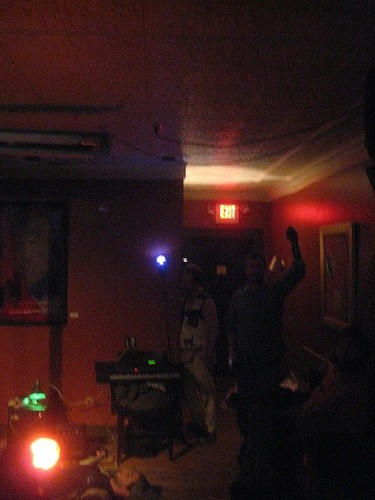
[[230, 226, 308, 466], [177, 262, 219, 438]]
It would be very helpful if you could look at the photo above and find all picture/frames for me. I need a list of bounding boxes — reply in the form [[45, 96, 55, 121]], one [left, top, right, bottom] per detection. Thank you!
[[317, 220, 357, 330]]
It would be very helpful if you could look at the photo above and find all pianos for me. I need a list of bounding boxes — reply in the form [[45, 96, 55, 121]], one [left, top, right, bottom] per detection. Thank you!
[[94, 350, 184, 436]]
